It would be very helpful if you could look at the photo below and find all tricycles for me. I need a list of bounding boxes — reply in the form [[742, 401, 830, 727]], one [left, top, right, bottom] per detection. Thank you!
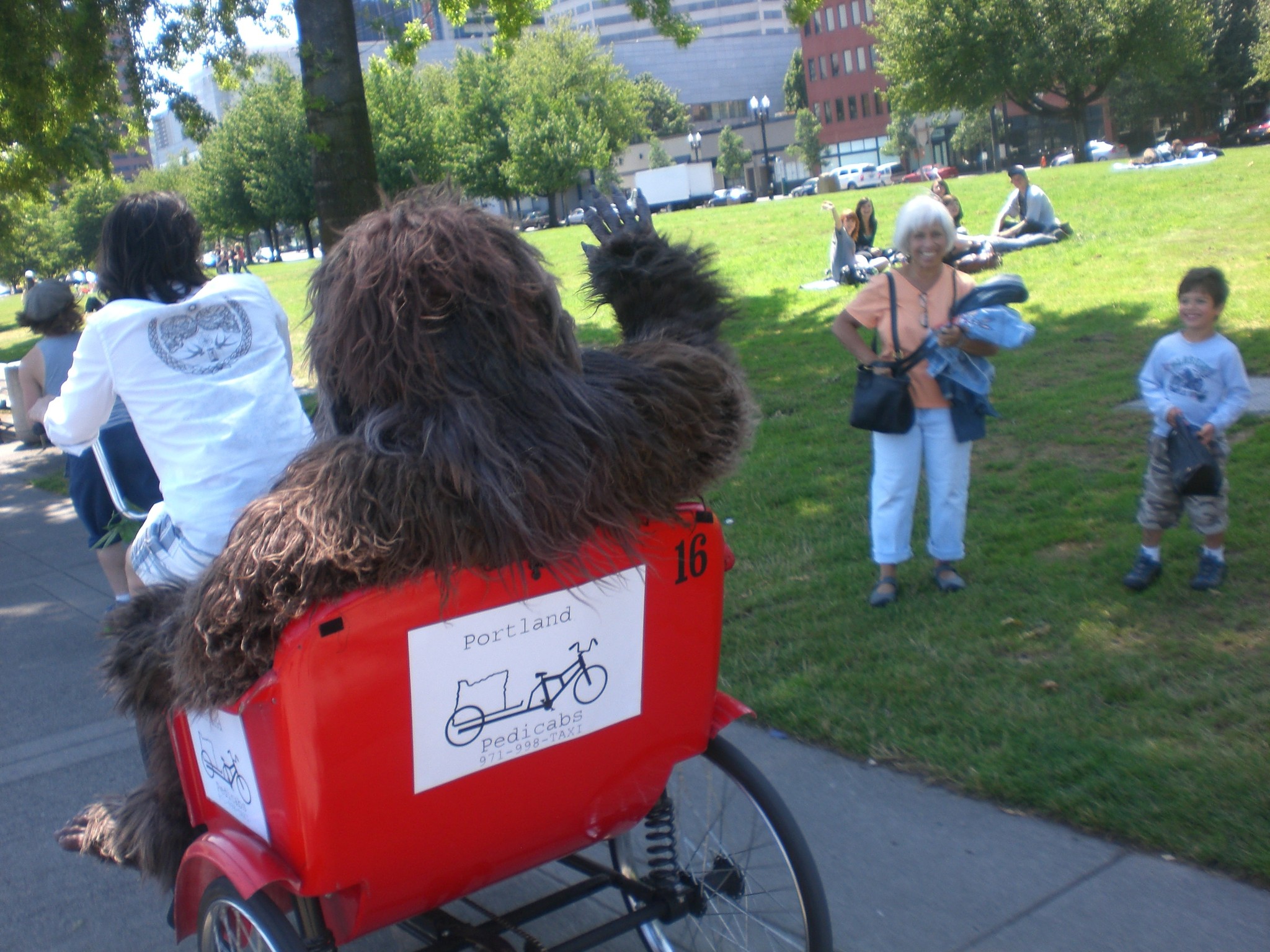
[[32, 411, 837, 952]]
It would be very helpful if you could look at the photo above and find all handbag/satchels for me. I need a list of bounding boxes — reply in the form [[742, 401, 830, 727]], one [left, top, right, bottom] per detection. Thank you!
[[852, 363, 919, 438], [1167, 414, 1225, 498]]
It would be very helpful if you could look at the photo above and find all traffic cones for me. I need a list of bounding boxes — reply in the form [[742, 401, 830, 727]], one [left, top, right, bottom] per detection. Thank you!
[[687, 131, 703, 163]]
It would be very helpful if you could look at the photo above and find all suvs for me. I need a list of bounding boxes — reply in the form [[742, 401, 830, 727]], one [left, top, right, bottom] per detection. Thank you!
[[814, 162, 882, 195], [519, 209, 559, 232], [876, 161, 907, 186]]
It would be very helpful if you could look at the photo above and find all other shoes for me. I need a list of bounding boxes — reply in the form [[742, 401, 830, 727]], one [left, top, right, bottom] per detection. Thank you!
[[933, 564, 966, 596], [102, 600, 130, 615], [868, 576, 902, 606]]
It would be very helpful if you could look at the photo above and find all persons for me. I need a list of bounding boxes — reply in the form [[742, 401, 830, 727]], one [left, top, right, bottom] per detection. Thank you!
[[994, 165, 1055, 239], [832, 195, 997, 604], [28, 189, 321, 598], [1122, 267, 1252, 590], [1131, 138, 1224, 166], [255, 246, 267, 263], [18, 278, 130, 617], [931, 180, 1069, 264], [83, 296, 107, 314], [214, 240, 251, 275], [1040, 153, 1047, 167], [21, 277, 38, 305], [821, 196, 889, 286]]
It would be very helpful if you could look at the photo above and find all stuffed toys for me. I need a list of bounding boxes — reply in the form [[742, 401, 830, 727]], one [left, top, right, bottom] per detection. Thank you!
[[57, 182, 758, 881]]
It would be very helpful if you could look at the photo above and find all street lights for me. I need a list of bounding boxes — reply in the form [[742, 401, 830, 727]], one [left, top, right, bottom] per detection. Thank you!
[[749, 92, 776, 201]]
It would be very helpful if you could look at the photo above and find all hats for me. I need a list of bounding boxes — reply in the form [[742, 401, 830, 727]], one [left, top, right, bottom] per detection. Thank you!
[[24, 270, 34, 278]]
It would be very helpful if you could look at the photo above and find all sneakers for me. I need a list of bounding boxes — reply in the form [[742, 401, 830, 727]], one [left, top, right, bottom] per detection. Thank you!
[[1189, 553, 1232, 590], [1125, 547, 1163, 590]]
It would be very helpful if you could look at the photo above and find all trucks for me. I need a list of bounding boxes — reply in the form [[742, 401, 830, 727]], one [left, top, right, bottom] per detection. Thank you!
[[625, 161, 715, 215]]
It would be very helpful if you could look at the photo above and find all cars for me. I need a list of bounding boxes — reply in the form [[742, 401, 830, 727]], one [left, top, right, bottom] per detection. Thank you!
[[790, 175, 819, 197], [706, 187, 757, 208], [900, 163, 959, 184], [1049, 137, 1130, 167], [1182, 113, 1270, 148], [565, 205, 598, 226]]
